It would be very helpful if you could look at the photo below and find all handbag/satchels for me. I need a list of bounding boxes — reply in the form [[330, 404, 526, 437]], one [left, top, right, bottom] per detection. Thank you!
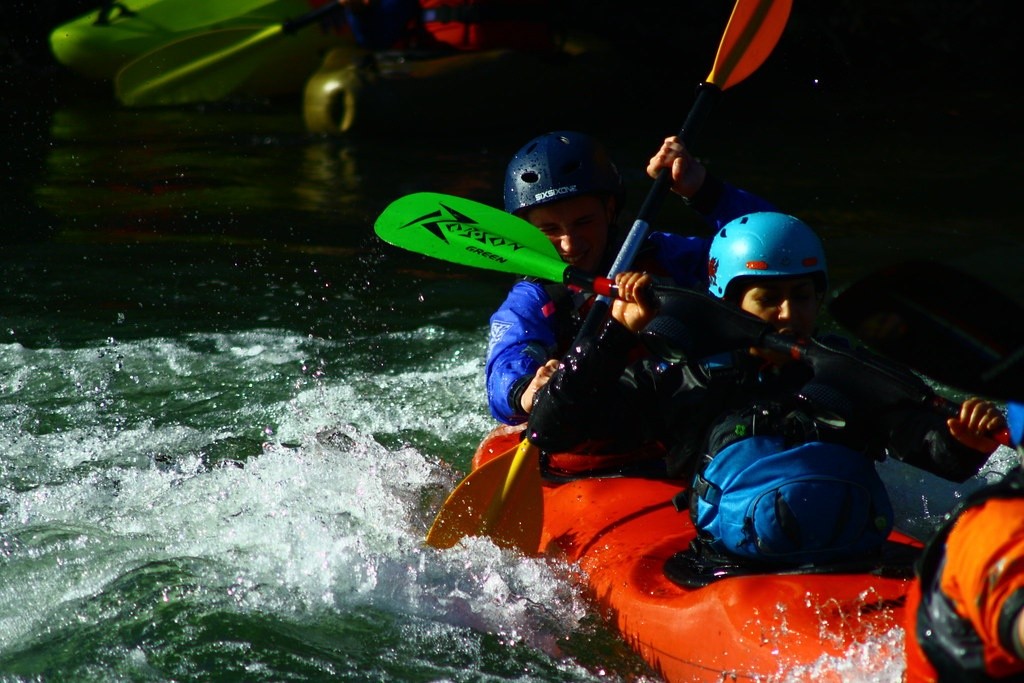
[[672, 409, 895, 575]]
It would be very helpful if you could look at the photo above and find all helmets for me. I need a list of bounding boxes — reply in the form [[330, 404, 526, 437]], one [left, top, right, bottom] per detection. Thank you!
[[708, 211, 829, 311], [503, 131, 613, 214]]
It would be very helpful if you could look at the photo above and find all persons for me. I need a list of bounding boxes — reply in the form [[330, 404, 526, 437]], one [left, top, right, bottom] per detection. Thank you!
[[327, 0, 548, 50], [486, 131, 1024, 683]]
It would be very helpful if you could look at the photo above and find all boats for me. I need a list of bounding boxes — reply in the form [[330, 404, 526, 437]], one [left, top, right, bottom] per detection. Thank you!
[[468, 418, 909, 683], [39, 0, 345, 105], [302, 30, 617, 139]]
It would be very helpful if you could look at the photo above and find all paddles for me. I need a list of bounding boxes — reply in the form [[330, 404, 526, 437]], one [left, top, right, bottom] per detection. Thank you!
[[116, 0, 339, 113], [376, 195, 964, 435], [426, 0, 794, 555]]
